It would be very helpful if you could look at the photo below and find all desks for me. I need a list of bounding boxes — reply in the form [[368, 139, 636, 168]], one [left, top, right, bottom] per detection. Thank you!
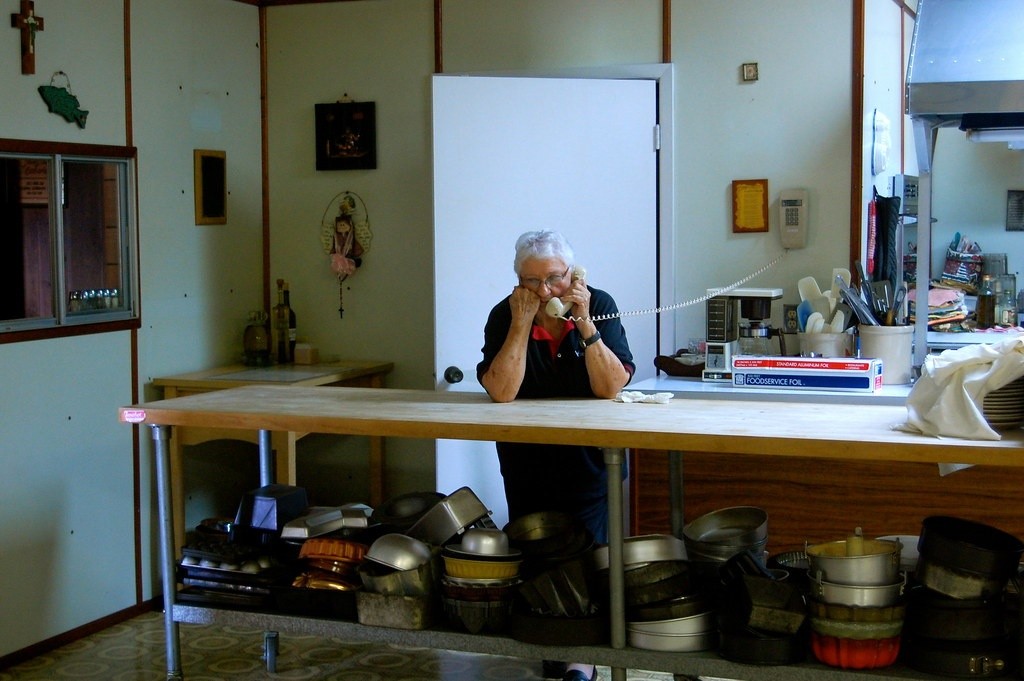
[[154, 363, 396, 590]]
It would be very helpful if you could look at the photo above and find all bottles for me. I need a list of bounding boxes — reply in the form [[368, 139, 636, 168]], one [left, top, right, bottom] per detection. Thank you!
[[69, 289, 118, 311], [243, 325, 270, 366], [273, 279, 290, 365], [977, 274, 996, 329], [283, 290, 296, 364], [999, 290, 1018, 328]]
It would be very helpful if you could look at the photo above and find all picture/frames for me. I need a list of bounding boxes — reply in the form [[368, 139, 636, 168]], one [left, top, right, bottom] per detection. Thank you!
[[193, 149, 228, 225], [315, 102, 378, 170]]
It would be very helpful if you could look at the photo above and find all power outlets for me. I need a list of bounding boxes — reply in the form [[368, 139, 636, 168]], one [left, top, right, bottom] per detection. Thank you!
[[782, 304, 798, 334]]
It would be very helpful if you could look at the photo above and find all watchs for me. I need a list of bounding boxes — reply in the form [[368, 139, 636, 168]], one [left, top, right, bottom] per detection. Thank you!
[[579, 332, 600, 348]]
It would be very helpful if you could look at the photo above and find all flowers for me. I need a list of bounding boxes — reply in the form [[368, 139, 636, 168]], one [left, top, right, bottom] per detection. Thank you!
[[328, 254, 349, 276]]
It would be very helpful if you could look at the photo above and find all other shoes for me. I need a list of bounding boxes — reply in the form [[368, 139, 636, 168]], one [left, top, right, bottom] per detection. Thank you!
[[562, 661, 596, 681]]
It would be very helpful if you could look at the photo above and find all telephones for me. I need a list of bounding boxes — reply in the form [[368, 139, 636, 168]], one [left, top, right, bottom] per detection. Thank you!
[[545, 264, 586, 318]]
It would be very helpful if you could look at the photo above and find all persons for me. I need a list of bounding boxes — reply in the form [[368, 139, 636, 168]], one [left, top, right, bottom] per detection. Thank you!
[[331, 215, 361, 269], [477, 229, 636, 681]]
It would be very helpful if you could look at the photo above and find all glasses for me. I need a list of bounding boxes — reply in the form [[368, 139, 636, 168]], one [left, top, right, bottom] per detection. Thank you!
[[519, 266, 570, 288]]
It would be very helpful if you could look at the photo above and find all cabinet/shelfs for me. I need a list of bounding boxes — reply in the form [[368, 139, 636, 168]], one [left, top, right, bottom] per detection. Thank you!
[[120, 385, 1024, 681]]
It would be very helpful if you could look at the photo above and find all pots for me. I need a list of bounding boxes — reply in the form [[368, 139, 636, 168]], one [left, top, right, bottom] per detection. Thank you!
[[281, 487, 1024, 676]]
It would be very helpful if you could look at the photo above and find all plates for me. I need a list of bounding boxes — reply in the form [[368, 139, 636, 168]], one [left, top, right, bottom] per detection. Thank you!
[[983, 375, 1024, 430]]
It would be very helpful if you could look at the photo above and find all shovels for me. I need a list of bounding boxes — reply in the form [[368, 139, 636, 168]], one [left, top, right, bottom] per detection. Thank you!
[[798, 276, 821, 302], [797, 299, 813, 332]]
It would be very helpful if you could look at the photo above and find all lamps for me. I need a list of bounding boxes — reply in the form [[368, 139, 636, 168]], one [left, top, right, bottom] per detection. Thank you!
[[966, 127, 1024, 142]]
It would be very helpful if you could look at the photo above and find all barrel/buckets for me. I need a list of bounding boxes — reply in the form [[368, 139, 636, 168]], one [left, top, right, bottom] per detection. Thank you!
[[798, 332, 854, 358], [857, 324, 914, 385]]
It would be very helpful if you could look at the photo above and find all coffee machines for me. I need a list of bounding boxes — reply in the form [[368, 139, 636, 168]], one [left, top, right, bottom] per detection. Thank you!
[[702, 287, 786, 381]]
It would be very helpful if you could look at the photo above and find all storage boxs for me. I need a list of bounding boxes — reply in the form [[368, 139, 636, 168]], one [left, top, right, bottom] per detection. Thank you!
[[731, 355, 882, 393], [355, 590, 431, 630], [740, 575, 806, 634], [267, 571, 366, 622]]
